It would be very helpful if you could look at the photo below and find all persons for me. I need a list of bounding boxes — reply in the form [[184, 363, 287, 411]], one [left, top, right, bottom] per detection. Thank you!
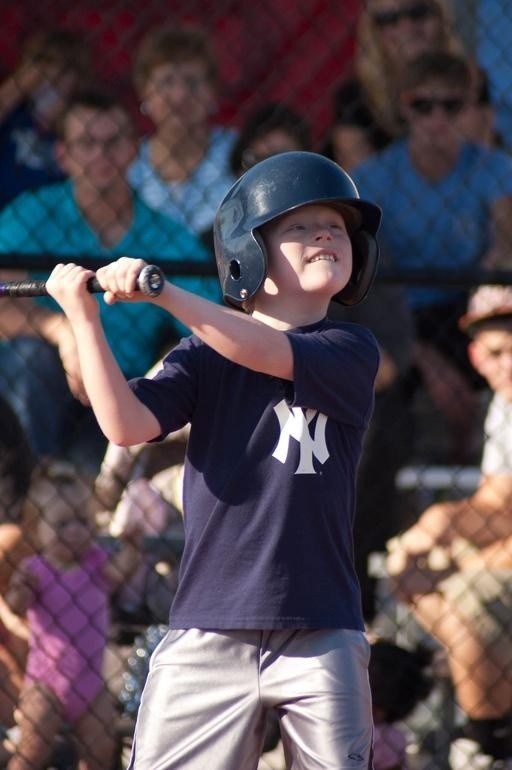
[[0, 0, 512, 770]]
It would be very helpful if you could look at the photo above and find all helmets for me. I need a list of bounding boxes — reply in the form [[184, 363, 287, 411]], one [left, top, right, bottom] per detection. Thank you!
[[212, 150, 384, 313]]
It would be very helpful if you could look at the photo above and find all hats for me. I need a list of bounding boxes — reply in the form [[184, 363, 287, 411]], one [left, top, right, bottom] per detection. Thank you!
[[458, 281, 512, 337]]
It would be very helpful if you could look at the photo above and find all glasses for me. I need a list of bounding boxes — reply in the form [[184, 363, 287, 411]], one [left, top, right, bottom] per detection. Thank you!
[[409, 97, 465, 116], [372, 4, 441, 25]]
